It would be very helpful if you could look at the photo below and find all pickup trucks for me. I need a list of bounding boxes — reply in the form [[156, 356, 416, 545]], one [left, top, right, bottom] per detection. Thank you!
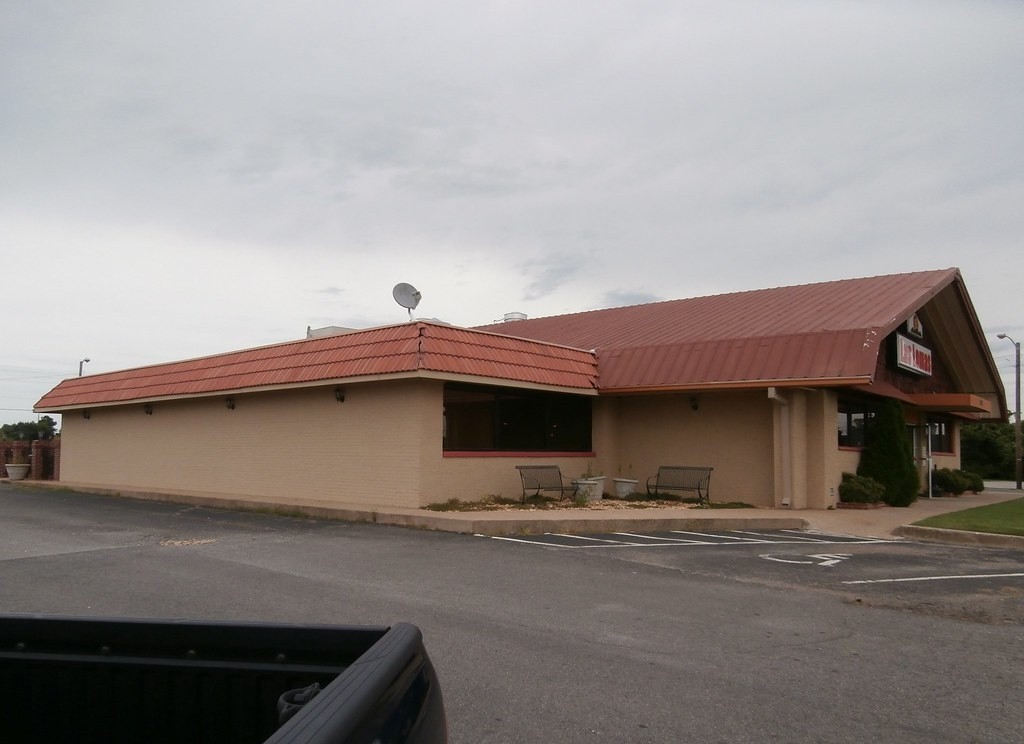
[[4, 617, 447, 744]]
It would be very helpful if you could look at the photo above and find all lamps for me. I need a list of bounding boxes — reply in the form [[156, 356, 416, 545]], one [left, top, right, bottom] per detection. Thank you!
[[83, 411, 89, 419], [334, 388, 344, 403], [225, 397, 235, 410], [144, 404, 152, 415]]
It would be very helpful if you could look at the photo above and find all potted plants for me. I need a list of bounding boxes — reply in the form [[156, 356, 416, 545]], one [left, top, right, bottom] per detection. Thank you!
[[613, 464, 638, 499], [571, 461, 607, 500], [4, 456, 31, 481]]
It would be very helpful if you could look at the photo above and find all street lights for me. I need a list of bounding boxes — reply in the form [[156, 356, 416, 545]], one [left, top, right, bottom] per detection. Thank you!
[[76, 356, 89, 372], [996, 332, 1022, 492]]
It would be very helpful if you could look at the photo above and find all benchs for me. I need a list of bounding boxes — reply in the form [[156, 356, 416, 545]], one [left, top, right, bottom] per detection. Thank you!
[[515, 465, 580, 505], [645, 466, 713, 504]]
